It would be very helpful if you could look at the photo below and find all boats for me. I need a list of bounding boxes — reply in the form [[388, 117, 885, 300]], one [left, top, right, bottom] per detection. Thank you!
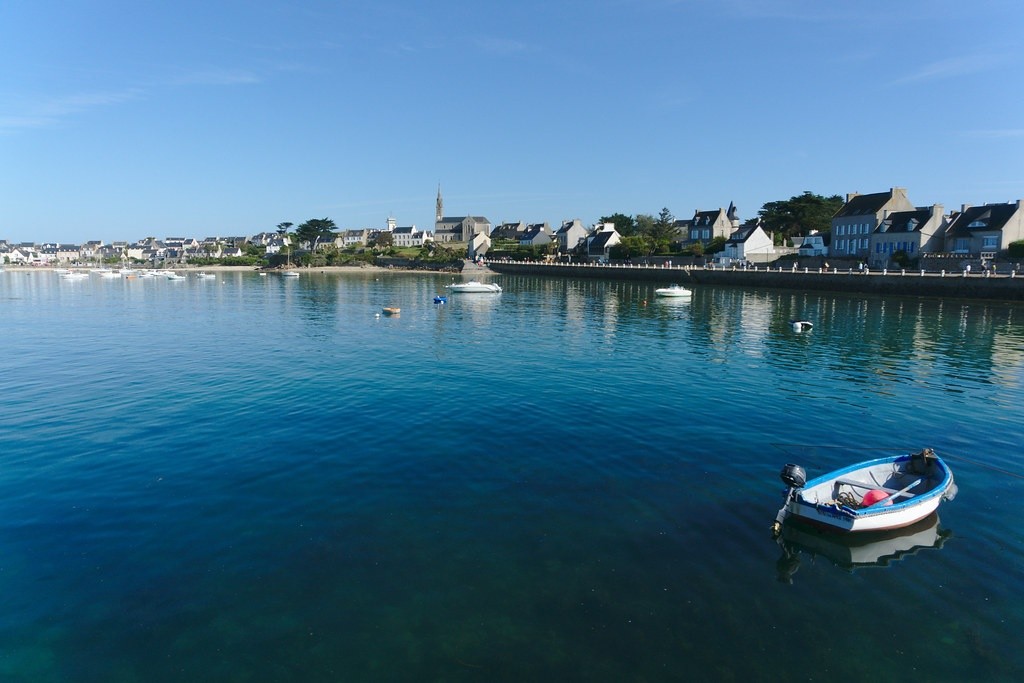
[[772, 445, 955, 537], [778, 510, 954, 572], [63, 249, 187, 279], [196, 270, 215, 278], [655, 283, 693, 297], [444, 279, 502, 292]]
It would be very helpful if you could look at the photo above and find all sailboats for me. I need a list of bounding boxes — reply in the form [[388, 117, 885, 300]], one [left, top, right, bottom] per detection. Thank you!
[[283, 249, 299, 276]]
